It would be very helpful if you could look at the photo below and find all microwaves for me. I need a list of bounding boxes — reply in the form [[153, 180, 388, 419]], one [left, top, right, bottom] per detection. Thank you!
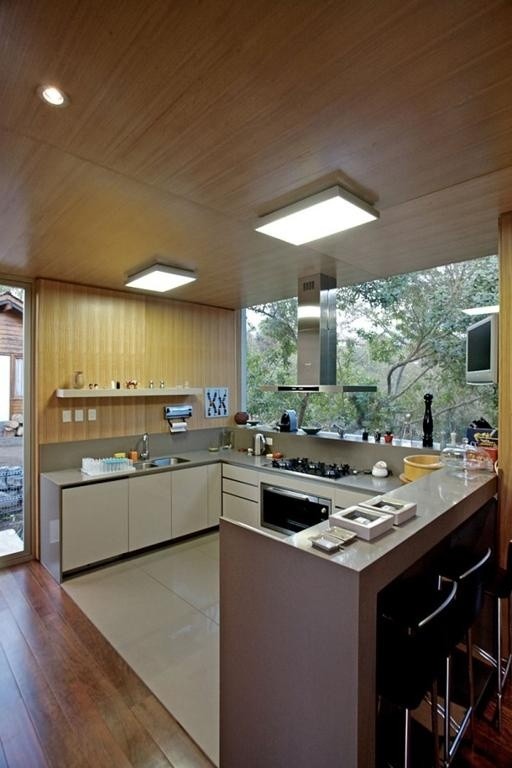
[[466, 314, 499, 385]]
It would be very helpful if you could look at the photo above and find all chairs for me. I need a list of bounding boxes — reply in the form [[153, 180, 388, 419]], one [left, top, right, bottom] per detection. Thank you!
[[377, 544, 511, 768]]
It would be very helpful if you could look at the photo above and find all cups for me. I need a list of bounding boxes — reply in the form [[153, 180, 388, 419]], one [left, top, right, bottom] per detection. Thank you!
[[82, 457, 134, 474]]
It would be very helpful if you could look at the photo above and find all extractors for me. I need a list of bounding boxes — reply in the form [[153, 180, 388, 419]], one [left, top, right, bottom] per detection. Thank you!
[[259, 274, 376, 393]]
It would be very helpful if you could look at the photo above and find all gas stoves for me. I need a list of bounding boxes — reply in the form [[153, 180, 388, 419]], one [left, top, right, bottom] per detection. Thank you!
[[262, 455, 354, 481]]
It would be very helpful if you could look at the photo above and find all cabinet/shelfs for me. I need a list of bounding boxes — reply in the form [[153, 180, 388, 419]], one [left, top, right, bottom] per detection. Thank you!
[[40, 460, 218, 584], [334, 470, 404, 513], [222, 446, 261, 531]]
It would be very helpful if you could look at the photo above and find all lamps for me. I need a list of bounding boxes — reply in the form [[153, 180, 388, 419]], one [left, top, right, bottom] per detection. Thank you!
[[253, 185, 380, 248], [125, 262, 197, 294]]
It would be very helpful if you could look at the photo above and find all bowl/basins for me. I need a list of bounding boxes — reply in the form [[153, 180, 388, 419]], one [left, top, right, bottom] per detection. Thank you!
[[246, 420, 260, 426], [303, 425, 321, 435], [466, 427, 498, 446], [402, 454, 442, 482]]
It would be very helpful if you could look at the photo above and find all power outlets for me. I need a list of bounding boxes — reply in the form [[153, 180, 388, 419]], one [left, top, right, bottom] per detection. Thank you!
[[75, 409, 84, 422], [87, 409, 97, 421], [63, 410, 72, 423]]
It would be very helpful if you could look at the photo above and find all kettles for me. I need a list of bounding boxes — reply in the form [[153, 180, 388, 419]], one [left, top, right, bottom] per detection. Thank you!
[[252, 433, 267, 457]]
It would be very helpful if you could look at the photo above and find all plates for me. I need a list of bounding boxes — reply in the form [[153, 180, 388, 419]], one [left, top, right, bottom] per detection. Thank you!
[[398, 472, 410, 484], [312, 526, 357, 552]]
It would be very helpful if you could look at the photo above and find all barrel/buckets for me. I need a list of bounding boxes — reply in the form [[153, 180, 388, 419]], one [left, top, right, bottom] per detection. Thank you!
[[140, 434, 149, 460]]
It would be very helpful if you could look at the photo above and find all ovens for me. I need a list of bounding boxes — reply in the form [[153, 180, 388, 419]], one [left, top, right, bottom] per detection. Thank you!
[[258, 482, 334, 537]]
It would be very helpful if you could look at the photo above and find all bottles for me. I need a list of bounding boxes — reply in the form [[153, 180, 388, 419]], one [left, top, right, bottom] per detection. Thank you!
[[89, 383, 98, 390], [148, 380, 153, 389], [437, 431, 469, 467], [70, 369, 84, 389], [362, 428, 368, 440], [384, 431, 393, 443], [159, 380, 164, 388], [374, 429, 381, 442], [423, 393, 434, 447]]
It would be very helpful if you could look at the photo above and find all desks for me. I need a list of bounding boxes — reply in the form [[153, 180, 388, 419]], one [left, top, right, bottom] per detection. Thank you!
[[219, 466, 496, 768]]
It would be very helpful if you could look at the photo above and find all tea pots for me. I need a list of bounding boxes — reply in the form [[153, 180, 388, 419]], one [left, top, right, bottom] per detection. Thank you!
[[370, 461, 393, 478]]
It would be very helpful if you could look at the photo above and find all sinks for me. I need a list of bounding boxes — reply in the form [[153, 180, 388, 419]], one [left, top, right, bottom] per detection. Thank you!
[[134, 462, 156, 470], [152, 457, 189, 467]]
